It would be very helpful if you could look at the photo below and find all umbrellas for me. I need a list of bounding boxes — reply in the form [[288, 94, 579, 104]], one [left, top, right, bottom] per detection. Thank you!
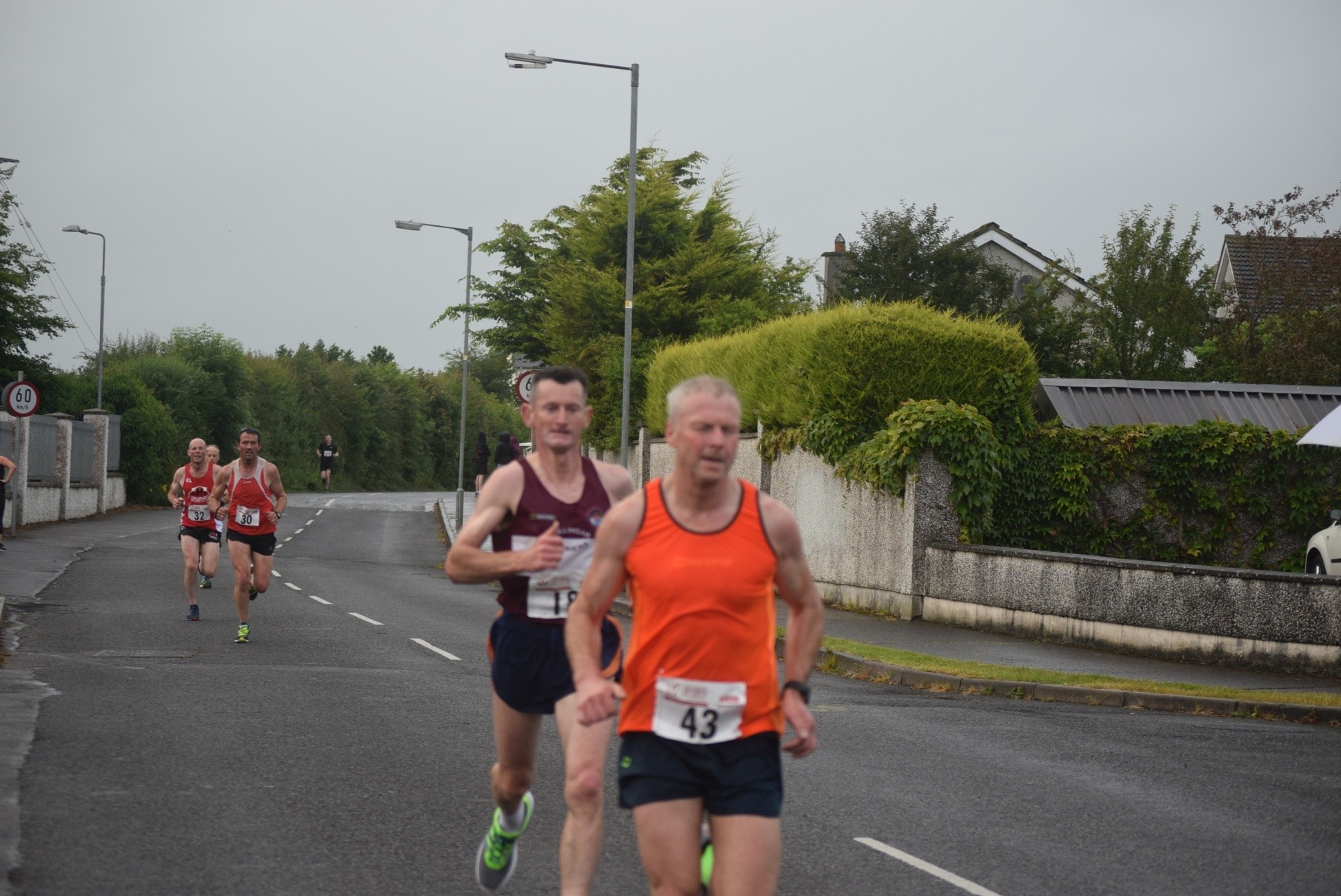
[[1295, 404, 1341, 448]]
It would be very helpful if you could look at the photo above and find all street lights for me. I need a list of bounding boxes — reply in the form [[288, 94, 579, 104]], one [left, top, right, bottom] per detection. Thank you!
[[393, 218, 474, 538], [61, 223, 105, 411], [502, 53, 641, 470]]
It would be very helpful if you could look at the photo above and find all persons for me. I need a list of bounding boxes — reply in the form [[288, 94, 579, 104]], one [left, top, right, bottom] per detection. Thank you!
[[444, 369, 637, 895], [316, 432, 339, 493], [474, 431, 492, 498], [199, 443, 230, 589], [494, 431, 521, 471], [206, 428, 286, 643], [167, 438, 224, 622], [0, 454, 17, 552], [570, 368, 825, 896]]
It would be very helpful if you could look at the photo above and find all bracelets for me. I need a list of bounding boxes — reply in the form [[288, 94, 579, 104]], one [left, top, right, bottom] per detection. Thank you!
[[214, 507, 220, 519]]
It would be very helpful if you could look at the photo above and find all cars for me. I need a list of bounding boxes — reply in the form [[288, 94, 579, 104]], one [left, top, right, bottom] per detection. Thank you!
[[1304, 507, 1341, 576]]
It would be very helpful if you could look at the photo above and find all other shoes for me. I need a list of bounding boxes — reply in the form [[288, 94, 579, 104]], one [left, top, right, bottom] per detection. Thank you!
[[699, 822, 715, 896], [475, 493, 479, 497], [0, 543, 7, 552]]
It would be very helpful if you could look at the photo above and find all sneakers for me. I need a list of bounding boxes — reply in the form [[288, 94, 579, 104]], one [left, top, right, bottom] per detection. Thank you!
[[200, 577, 212, 588], [187, 605, 201, 621], [248, 563, 259, 600], [474, 790, 535, 896], [198, 553, 205, 576], [233, 623, 251, 643]]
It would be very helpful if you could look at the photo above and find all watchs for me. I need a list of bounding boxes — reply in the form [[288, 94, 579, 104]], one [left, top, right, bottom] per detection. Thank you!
[[220, 499, 225, 507], [780, 681, 810, 705], [274, 511, 281, 519]]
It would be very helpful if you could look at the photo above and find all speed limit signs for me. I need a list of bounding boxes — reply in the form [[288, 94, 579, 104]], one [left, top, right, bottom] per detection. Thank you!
[[514, 370, 540, 404], [6, 381, 41, 420]]
